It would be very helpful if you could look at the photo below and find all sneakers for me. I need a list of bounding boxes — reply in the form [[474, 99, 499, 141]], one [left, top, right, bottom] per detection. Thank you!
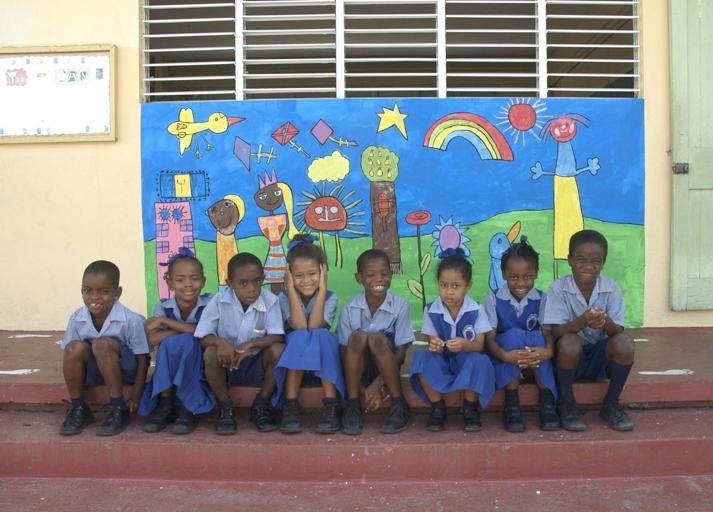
[[172, 404, 200, 434], [503, 403, 525, 432], [599, 398, 634, 431], [426, 401, 447, 431], [99, 403, 132, 436], [215, 396, 242, 435], [142, 399, 178, 433], [462, 404, 482, 432], [250, 403, 280, 432], [538, 399, 587, 431], [60, 398, 95, 436], [314, 400, 364, 435], [380, 399, 412, 434], [280, 399, 303, 433]]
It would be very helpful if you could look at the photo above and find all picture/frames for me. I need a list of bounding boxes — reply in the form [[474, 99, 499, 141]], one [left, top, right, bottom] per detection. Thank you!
[[0, 42, 118, 141]]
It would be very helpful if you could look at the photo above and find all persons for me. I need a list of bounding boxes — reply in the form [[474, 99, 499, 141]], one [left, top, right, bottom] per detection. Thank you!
[[271, 233, 346, 433], [406, 254, 495, 433], [483, 242, 562, 431], [193, 252, 286, 435], [337, 249, 414, 434], [59, 259, 151, 436], [138, 247, 218, 434], [540, 230, 635, 430]]
